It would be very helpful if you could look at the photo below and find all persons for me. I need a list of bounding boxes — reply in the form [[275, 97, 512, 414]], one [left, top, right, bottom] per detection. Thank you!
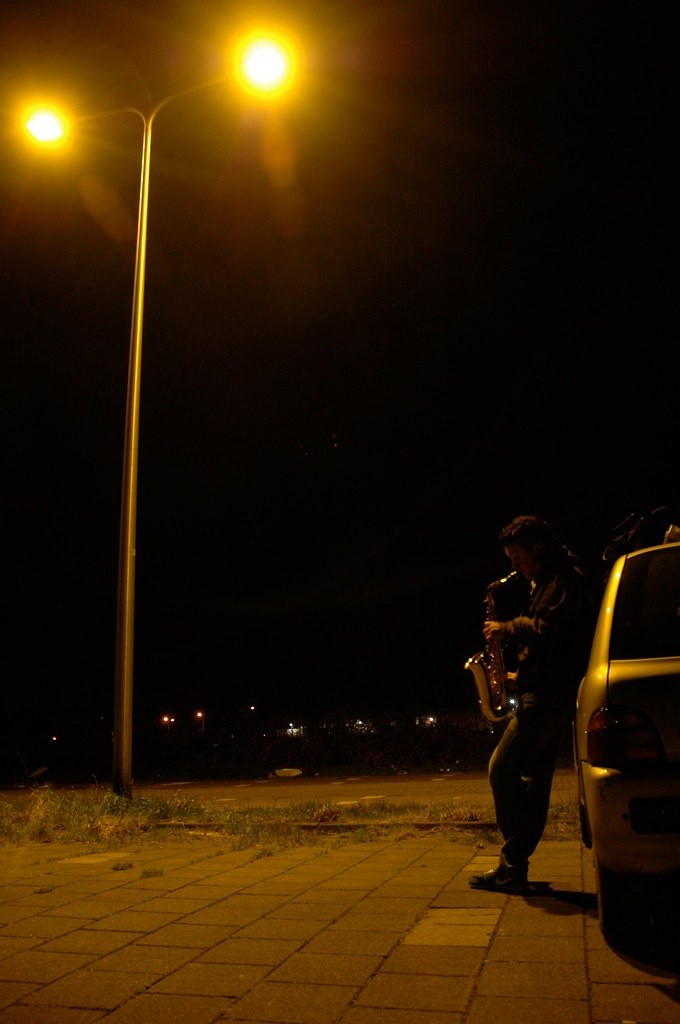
[[468, 515, 597, 892]]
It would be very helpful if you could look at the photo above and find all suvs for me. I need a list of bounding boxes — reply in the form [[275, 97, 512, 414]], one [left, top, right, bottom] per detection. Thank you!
[[573, 539, 680, 953]]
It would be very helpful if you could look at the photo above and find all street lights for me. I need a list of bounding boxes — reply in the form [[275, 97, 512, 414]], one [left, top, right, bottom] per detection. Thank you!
[[22, 50, 282, 802]]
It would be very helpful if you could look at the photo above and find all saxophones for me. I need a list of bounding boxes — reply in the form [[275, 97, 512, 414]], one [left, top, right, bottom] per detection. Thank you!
[[463, 569, 522, 725]]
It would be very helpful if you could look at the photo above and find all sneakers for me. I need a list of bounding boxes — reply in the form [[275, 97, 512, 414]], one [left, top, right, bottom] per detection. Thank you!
[[471, 863, 528, 894]]
